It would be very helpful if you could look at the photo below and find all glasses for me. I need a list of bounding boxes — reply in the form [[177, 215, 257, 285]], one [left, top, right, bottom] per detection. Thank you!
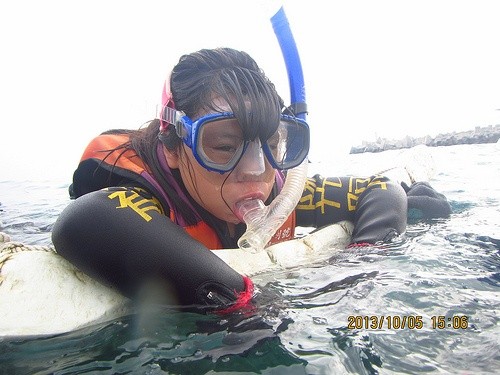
[[174, 110, 310, 175]]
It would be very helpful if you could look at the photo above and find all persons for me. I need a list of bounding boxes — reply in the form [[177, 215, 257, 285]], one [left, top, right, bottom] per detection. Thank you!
[[51, 47, 408, 314]]
